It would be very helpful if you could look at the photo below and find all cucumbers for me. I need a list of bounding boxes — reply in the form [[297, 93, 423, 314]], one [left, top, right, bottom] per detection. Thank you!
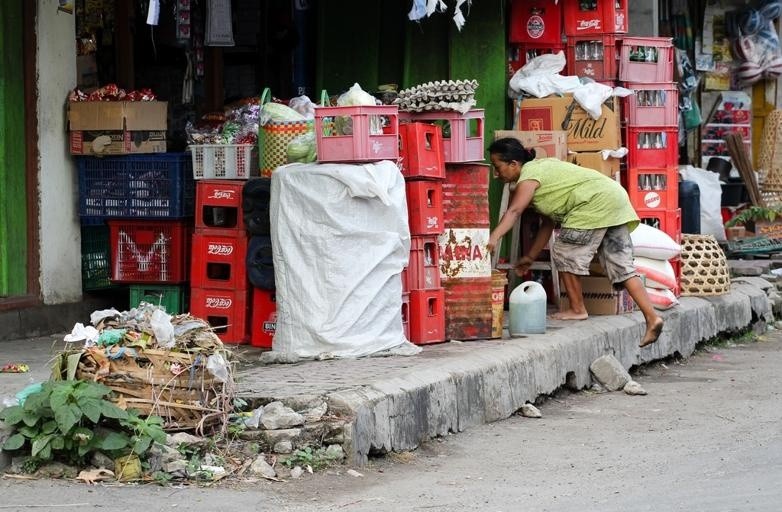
[[286, 140, 316, 163]]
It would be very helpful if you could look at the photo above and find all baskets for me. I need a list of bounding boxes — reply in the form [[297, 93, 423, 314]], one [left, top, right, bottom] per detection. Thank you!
[[77, 87, 485, 433], [189, 143, 255, 179], [509, 0, 730, 298], [758, 109, 781, 206], [679, 233, 731, 296]]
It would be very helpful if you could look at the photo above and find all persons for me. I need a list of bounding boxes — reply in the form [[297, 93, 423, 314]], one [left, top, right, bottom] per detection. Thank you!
[[486, 138, 663, 347]]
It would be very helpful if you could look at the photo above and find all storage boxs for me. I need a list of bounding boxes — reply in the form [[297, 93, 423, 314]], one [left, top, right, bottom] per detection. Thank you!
[[492, 95, 624, 316], [64, 99, 168, 157]]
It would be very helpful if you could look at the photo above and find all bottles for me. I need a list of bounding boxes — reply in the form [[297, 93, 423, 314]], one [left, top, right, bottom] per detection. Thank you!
[[629, 47, 657, 63], [642, 218, 659, 229], [513, 47, 545, 67], [576, 41, 604, 60], [425, 245, 433, 265], [637, 132, 666, 149], [426, 135, 431, 150], [638, 174, 665, 190], [580, 1, 596, 10], [637, 92, 663, 106]]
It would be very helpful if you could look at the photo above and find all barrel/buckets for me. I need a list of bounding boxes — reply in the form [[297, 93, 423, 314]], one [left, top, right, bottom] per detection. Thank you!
[[506, 279, 548, 338]]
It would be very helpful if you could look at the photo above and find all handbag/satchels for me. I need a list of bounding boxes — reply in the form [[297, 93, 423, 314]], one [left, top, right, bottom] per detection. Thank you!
[[258, 87, 332, 178]]
